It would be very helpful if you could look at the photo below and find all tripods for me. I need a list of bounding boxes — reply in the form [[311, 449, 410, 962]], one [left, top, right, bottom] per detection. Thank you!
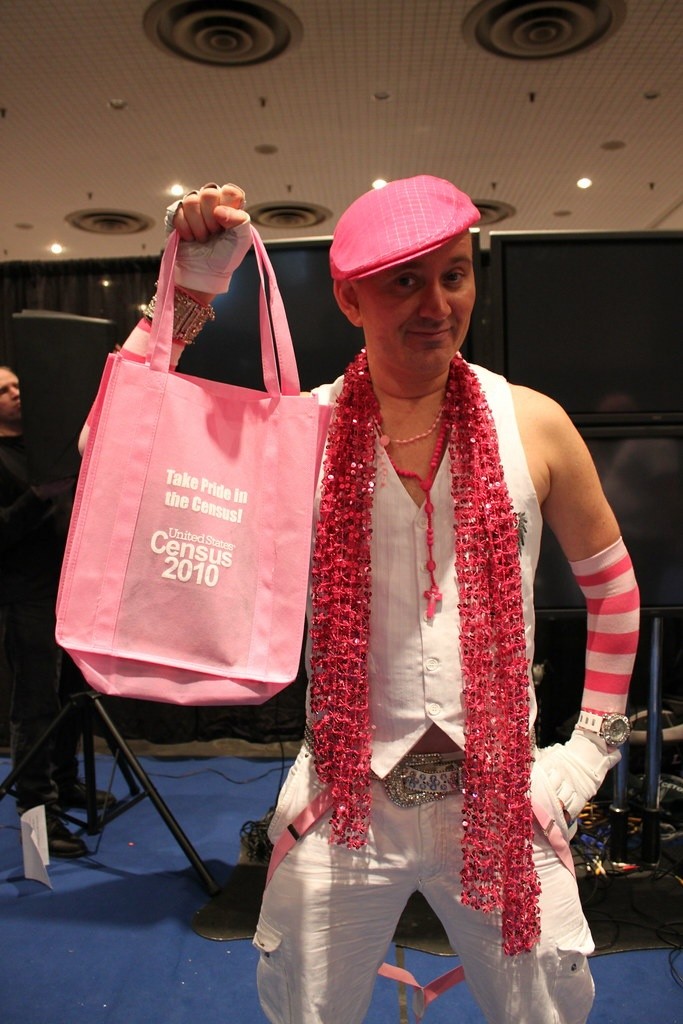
[[0, 674, 221, 897]]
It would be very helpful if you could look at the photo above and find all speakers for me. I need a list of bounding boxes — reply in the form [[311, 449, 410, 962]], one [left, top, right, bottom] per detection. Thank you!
[[10, 309, 120, 486]]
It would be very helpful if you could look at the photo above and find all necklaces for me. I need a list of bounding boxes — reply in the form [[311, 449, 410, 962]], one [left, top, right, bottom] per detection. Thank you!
[[372, 403, 448, 446], [372, 408, 450, 619]]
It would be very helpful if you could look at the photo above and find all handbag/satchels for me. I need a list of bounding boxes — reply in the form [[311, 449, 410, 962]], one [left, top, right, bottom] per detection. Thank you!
[[54, 220, 336, 709]]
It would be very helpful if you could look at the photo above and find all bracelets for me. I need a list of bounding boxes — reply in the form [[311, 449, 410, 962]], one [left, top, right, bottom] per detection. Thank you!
[[141, 281, 215, 345]]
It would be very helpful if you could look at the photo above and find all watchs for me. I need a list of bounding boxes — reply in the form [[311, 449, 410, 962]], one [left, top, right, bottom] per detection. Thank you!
[[575, 711, 631, 747]]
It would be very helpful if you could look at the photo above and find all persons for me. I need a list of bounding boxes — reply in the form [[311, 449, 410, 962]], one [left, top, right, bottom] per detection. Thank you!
[[0, 365, 117, 856], [77, 176, 640, 1024]]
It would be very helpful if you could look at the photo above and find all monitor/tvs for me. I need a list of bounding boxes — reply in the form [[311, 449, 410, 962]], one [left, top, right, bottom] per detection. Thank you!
[[178, 230, 683, 623]]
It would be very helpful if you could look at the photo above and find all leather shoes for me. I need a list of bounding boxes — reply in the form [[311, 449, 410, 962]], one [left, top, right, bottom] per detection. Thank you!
[[18, 812, 89, 857], [49, 778, 117, 811]]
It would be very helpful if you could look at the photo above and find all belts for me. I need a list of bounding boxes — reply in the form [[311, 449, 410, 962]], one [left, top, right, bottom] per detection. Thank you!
[[303, 719, 468, 809]]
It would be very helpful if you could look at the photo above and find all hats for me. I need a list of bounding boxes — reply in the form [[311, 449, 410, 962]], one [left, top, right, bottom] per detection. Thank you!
[[328, 172, 481, 284]]
